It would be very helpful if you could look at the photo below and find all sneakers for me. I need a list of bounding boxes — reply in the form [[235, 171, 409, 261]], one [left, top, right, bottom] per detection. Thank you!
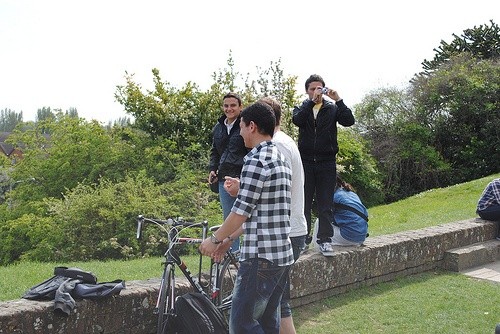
[[300, 243, 309, 254], [319, 242, 335, 257]]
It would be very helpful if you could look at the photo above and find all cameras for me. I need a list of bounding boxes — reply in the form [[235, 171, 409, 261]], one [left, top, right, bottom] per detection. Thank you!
[[319, 88, 327, 94]]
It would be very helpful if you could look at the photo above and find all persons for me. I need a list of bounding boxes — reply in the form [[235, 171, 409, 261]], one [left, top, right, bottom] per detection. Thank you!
[[223, 97, 307, 334], [476, 178, 500, 240], [199, 101, 295, 334], [292, 74, 355, 256], [311, 176, 368, 252], [209, 93, 253, 265]]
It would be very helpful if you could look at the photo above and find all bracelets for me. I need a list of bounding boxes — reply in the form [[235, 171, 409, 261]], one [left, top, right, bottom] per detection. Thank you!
[[228, 236, 233, 241]]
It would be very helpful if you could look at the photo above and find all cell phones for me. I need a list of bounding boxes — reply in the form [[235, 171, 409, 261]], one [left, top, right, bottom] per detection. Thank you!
[[210, 174, 218, 182]]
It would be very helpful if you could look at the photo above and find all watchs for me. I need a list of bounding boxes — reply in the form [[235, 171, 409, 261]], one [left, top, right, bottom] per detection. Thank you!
[[211, 232, 223, 244]]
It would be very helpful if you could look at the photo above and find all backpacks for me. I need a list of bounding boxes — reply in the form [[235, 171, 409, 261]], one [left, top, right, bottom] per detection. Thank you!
[[166, 293, 229, 334]]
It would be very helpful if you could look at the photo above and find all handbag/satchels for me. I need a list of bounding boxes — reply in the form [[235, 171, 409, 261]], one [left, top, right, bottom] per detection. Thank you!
[[210, 174, 219, 193]]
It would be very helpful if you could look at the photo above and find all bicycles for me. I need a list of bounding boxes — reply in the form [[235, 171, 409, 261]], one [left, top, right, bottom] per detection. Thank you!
[[135, 213, 241, 334]]
